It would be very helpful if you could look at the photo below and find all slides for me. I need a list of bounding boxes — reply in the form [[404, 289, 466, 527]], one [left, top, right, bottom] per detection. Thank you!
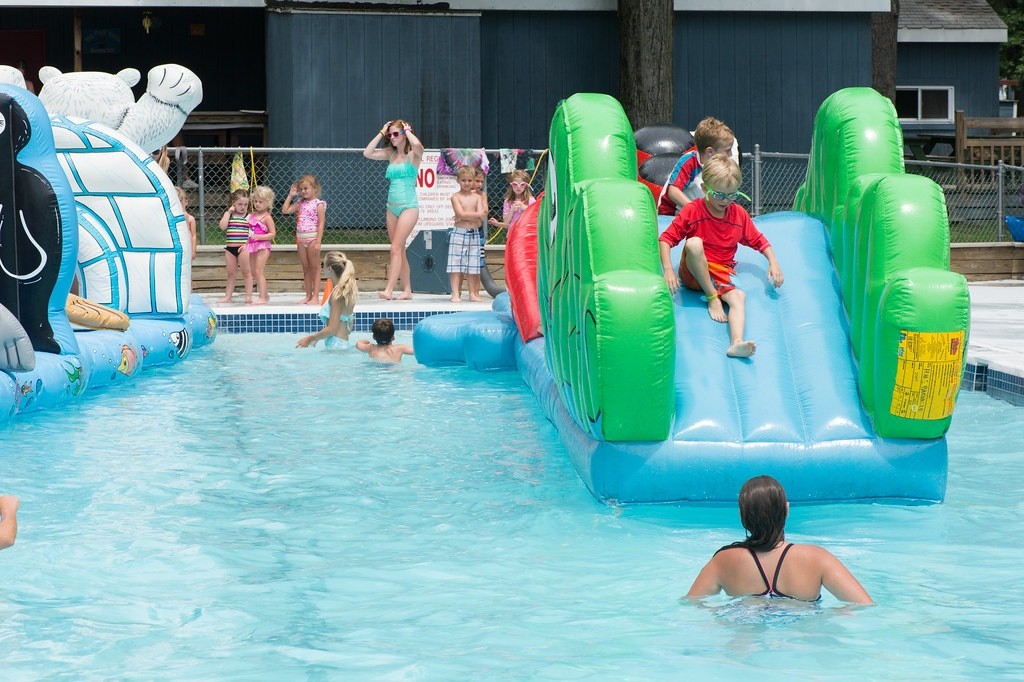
[[592, 209, 973, 503]]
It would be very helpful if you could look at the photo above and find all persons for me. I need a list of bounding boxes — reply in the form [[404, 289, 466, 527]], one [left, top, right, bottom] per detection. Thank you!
[[149, 143, 170, 174], [444, 163, 489, 302], [687, 475, 873, 603], [362, 118, 424, 298], [218, 187, 253, 304], [294, 250, 357, 349], [245, 185, 276, 306], [658, 153, 784, 358], [0, 494, 20, 551], [657, 116, 735, 216], [489, 170, 537, 239], [355, 317, 415, 363], [281, 175, 326, 304], [173, 187, 197, 260]]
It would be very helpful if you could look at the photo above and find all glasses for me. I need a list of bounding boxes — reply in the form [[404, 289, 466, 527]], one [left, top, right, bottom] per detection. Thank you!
[[152, 150, 161, 155], [510, 182, 526, 188], [704, 183, 738, 200], [321, 262, 329, 269], [387, 130, 404, 138]]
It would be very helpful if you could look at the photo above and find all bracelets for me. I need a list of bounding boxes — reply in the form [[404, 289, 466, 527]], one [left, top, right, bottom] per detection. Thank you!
[[379, 129, 385, 136], [403, 128, 410, 134]]
